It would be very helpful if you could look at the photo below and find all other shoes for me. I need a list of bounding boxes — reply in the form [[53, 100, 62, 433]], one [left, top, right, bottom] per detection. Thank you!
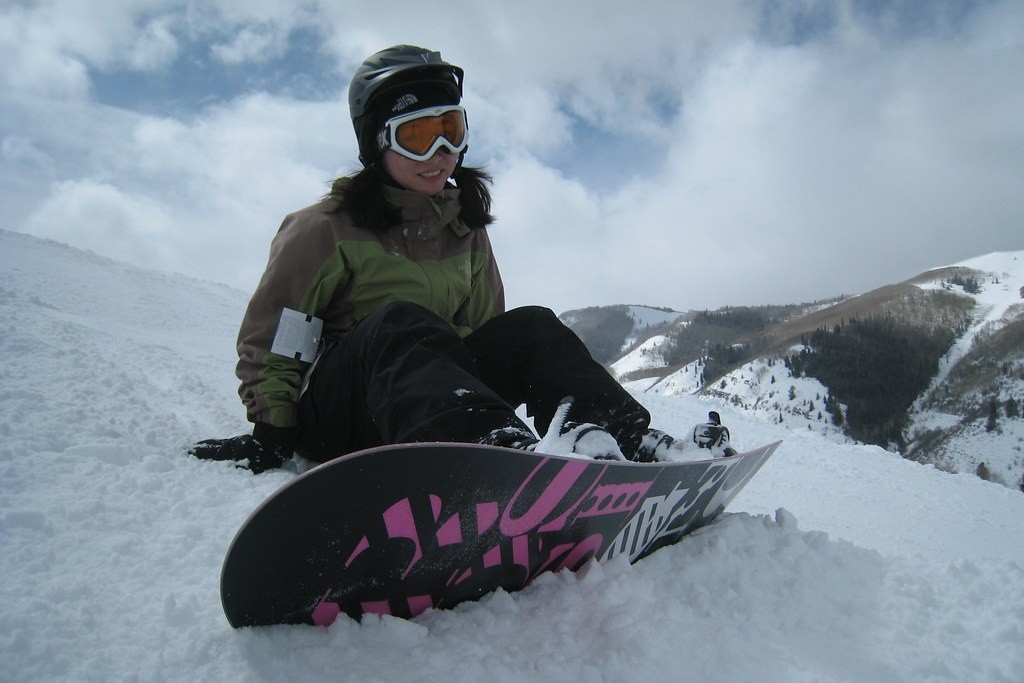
[[471, 396, 740, 462]]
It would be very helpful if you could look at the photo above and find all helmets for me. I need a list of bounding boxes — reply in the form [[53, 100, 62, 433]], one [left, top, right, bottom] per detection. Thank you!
[[348, 43, 470, 169]]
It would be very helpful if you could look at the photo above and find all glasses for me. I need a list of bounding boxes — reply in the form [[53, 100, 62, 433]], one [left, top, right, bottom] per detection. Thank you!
[[381, 104, 471, 160]]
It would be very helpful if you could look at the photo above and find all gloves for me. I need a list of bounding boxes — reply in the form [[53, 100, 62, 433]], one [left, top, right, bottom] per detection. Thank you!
[[187, 432, 297, 474]]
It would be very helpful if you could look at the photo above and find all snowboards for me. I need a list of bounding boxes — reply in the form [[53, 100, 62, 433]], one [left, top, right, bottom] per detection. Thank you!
[[219, 438, 785, 630]]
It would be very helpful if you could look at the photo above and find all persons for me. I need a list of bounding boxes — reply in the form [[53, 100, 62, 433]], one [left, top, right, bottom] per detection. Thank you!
[[187, 44, 738, 475]]
